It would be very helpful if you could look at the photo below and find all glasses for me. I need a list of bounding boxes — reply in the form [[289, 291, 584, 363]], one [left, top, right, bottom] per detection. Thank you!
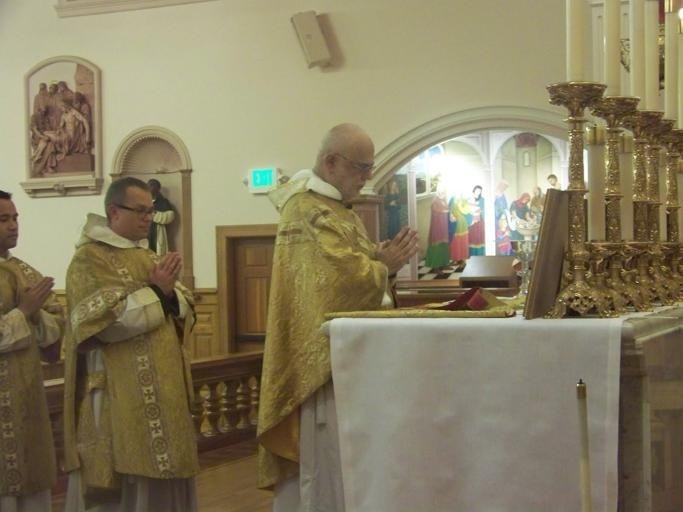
[[111, 202, 156, 219], [334, 152, 376, 174]]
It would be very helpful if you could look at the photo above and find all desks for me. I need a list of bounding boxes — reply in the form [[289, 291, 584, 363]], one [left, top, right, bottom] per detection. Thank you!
[[321, 308, 683, 512]]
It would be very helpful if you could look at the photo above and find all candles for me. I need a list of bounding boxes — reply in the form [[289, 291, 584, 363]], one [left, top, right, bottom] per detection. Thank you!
[[619, 153, 634, 241], [658, 168, 667, 241], [576, 379, 592, 512], [678, 33, 683, 129], [566, 0, 584, 82], [646, 2, 658, 111], [588, 145, 605, 242], [604, 0, 621, 97], [629, 0, 646, 110], [676, 173, 683, 242], [664, 12, 678, 120]]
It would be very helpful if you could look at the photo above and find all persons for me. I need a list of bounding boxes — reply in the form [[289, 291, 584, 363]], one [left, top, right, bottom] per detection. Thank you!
[[146, 178, 176, 259], [387, 172, 563, 275], [30, 81, 93, 179], [62, 178, 201, 512], [255, 121, 420, 512], [0, 191, 65, 512]]
